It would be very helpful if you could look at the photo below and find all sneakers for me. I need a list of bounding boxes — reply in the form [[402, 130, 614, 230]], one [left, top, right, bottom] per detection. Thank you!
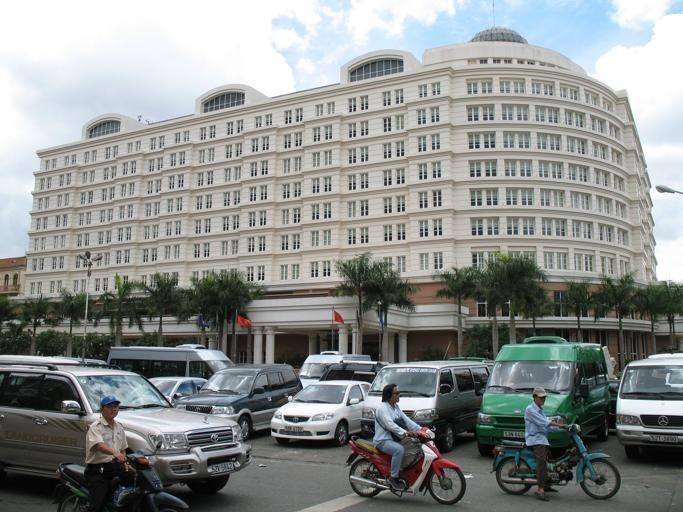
[[535, 490, 551, 503], [544, 486, 560, 493], [386, 476, 408, 492]]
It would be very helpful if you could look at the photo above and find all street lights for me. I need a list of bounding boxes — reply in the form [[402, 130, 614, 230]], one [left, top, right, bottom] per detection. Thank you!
[[653, 185, 682, 196], [76, 251, 102, 363]]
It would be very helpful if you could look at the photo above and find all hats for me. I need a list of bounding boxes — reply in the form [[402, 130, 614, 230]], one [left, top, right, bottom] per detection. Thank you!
[[98, 395, 122, 407], [532, 386, 548, 398]]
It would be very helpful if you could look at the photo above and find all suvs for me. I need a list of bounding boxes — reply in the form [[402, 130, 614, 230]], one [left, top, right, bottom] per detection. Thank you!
[[0, 354, 253, 499]]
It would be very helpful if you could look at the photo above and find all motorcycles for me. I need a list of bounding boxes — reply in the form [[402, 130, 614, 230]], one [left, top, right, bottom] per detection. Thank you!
[[54, 441, 189, 512], [490, 415, 622, 500], [344, 420, 467, 504]]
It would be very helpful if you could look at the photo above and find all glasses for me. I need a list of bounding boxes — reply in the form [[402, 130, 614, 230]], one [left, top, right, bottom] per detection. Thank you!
[[391, 391, 400, 395]]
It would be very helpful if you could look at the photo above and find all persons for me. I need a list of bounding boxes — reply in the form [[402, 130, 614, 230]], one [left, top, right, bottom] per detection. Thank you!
[[83, 395, 137, 512], [372, 384, 422, 491], [524, 387, 571, 501]]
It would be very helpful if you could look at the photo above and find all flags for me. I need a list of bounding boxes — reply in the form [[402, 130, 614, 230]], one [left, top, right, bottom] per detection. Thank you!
[[236, 313, 252, 327], [199, 313, 211, 328], [378, 305, 385, 332], [333, 310, 344, 324]]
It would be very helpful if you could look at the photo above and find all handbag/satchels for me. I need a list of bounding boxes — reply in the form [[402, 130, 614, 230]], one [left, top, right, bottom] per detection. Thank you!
[[390, 416, 408, 442]]
